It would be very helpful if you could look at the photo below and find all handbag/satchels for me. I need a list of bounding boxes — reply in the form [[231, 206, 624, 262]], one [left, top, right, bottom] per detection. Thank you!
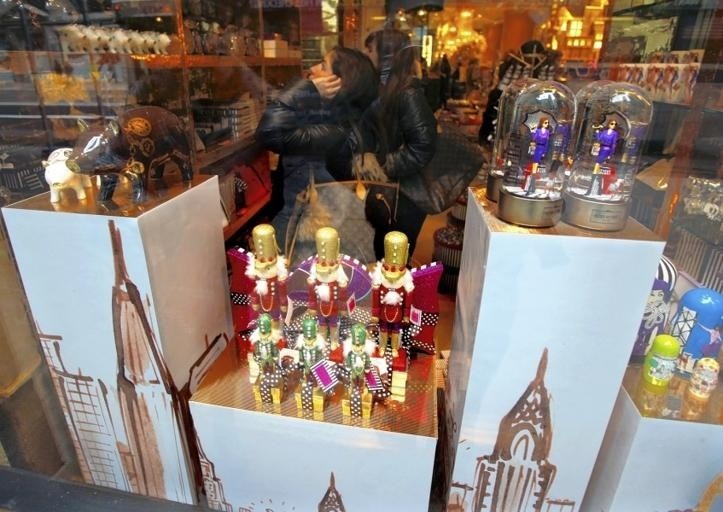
[[285, 182, 380, 273], [389, 118, 485, 217]]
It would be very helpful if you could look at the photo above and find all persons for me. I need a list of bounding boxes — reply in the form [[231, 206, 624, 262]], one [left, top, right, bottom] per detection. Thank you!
[[362, 29, 438, 270], [544, 47, 564, 83], [478, 40, 549, 153], [420, 52, 477, 88], [522, 115, 550, 193], [583, 117, 619, 196], [498, 49, 516, 80], [208, 43, 381, 256]]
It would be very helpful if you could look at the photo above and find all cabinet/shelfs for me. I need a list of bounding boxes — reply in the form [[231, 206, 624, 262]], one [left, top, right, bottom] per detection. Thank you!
[[1, 1, 303, 246], [597, 0, 722, 292]]
